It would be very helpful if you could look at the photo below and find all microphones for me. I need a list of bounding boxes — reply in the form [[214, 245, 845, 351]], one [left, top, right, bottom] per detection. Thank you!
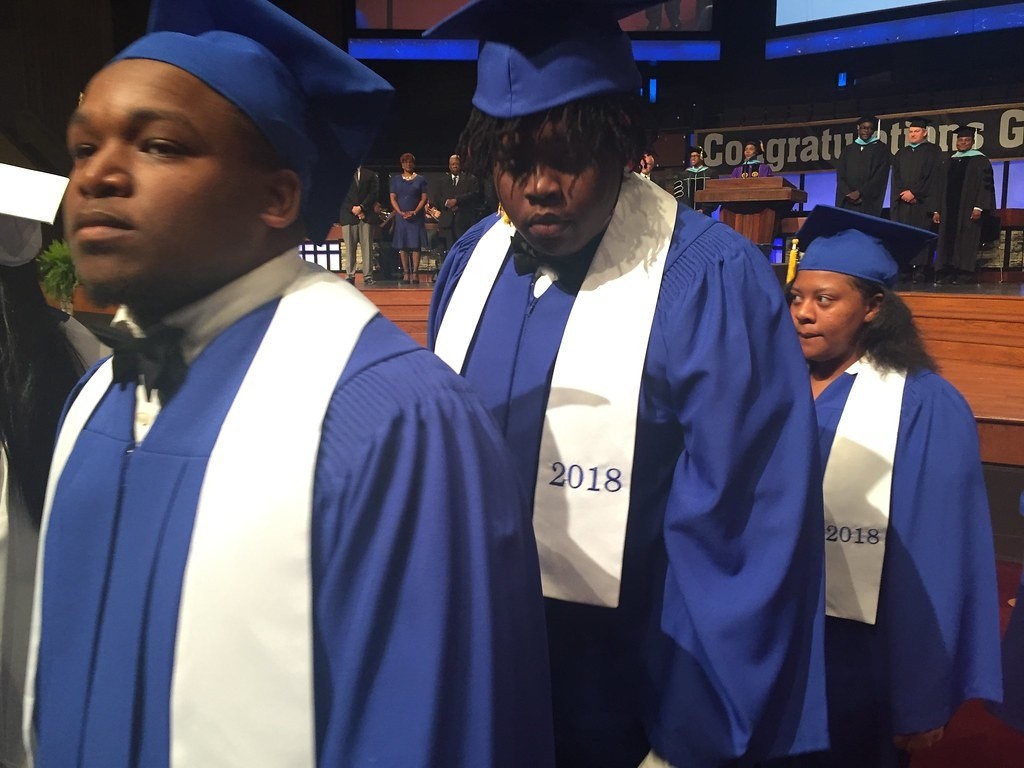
[[746, 150, 763, 161]]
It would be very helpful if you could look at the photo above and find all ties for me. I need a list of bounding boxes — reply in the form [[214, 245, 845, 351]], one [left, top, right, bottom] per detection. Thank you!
[[453, 176, 457, 187]]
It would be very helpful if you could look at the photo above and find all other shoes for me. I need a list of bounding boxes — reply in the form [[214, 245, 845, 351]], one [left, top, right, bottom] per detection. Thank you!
[[412, 271, 420, 284], [402, 270, 411, 284], [952, 273, 974, 285], [346, 278, 355, 283], [366, 278, 376, 284]]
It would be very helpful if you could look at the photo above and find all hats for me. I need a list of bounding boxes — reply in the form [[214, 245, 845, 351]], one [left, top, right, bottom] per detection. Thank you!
[[951, 126, 981, 149], [742, 136, 766, 152], [900, 116, 933, 147], [786, 204, 939, 285], [688, 145, 707, 161], [107, 0, 397, 247], [421, 0, 664, 119], [856, 116, 881, 139], [0, 162, 71, 267]]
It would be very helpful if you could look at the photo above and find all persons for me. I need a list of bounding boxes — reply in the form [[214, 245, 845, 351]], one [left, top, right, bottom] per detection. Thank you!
[[783, 205, 1005, 768], [422, 0, 830, 768], [339, 113, 999, 286], [0, 0, 556, 768]]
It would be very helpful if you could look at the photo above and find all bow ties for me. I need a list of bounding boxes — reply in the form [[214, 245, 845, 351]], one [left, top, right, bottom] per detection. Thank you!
[[94, 323, 186, 402], [511, 236, 572, 288]]
